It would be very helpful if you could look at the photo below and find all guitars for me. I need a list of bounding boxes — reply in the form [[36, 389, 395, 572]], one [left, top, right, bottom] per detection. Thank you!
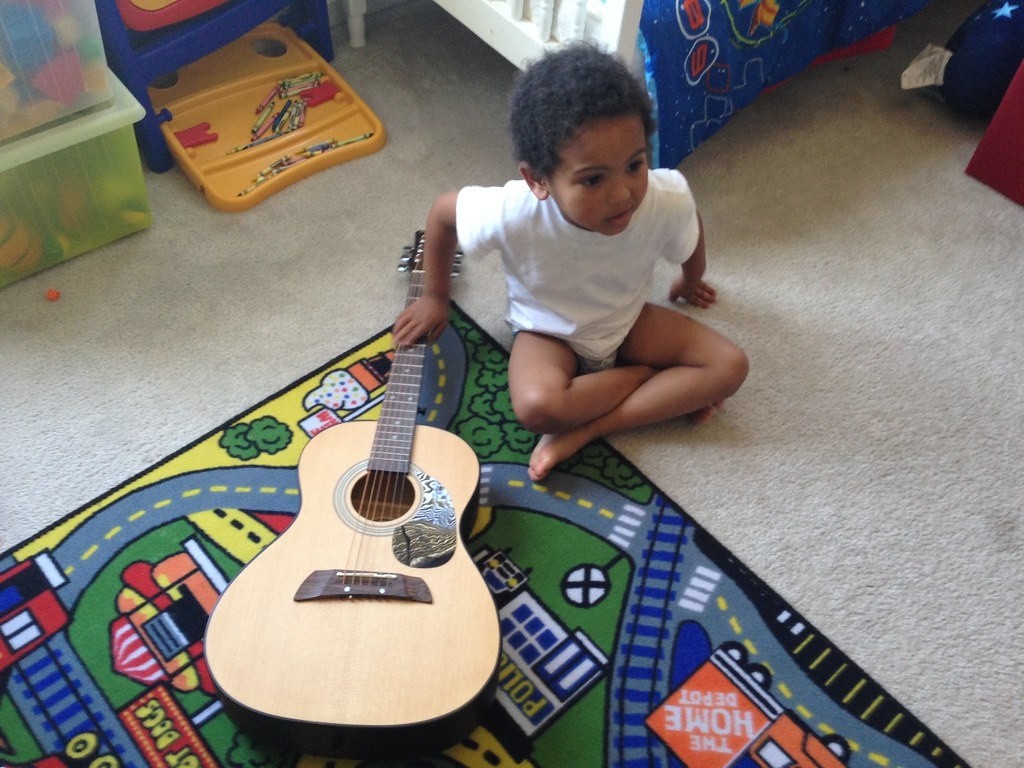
[[202, 230, 501, 747]]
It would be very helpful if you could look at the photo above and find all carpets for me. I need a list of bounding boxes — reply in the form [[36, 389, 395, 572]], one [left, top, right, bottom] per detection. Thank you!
[[0, 299, 972, 768]]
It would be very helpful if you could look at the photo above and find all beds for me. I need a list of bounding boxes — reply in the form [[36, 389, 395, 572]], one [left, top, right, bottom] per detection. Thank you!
[[346, 0, 930, 170]]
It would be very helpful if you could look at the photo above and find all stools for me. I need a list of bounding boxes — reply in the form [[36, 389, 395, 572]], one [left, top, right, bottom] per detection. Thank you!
[[95, 0, 335, 174]]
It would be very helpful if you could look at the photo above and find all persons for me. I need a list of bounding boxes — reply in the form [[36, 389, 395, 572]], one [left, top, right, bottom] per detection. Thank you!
[[393, 44, 751, 481]]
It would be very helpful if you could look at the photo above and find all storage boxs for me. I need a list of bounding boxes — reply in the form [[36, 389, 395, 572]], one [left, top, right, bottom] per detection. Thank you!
[[0, 67, 151, 290], [0, 0, 114, 141]]
